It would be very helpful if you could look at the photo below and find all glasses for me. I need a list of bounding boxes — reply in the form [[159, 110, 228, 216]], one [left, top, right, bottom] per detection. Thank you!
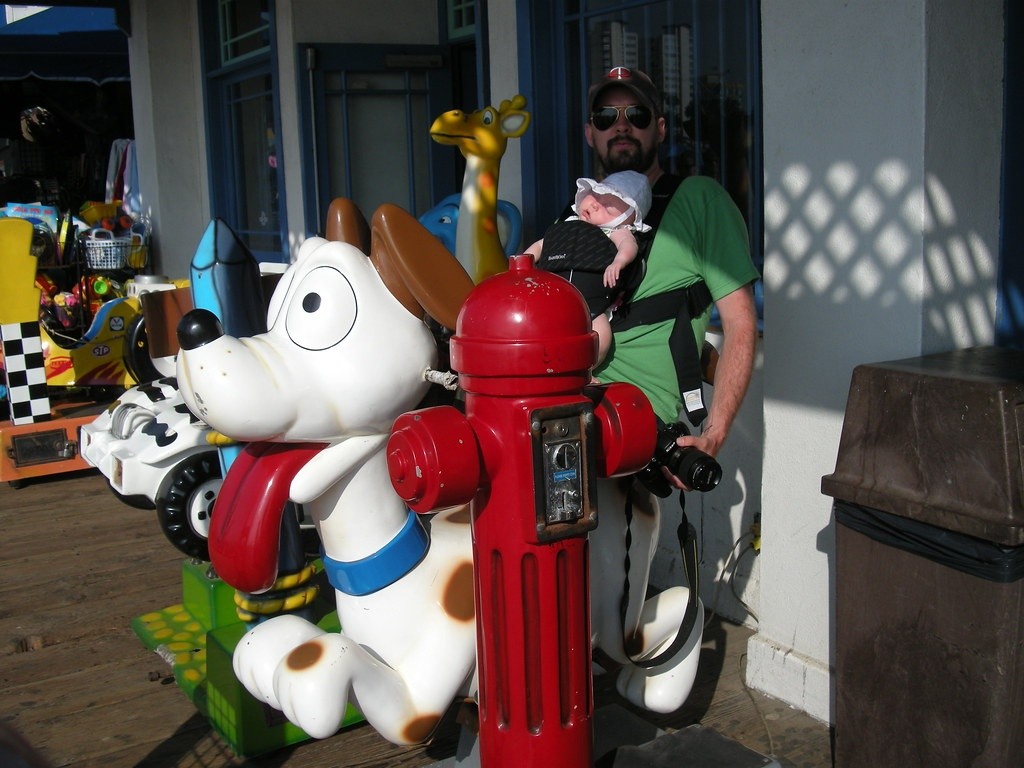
[[588, 105, 653, 131]]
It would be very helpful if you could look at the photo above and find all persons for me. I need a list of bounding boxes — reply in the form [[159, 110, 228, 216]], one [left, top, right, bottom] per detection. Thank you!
[[0, 148, 32, 207], [553, 66, 761, 492], [523, 171, 653, 384]]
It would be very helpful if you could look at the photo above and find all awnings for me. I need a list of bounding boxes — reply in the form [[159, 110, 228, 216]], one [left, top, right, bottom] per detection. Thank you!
[[0, 6, 133, 86]]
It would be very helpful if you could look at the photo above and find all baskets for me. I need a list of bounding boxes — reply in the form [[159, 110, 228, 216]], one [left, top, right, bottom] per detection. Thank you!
[[83, 224, 133, 271]]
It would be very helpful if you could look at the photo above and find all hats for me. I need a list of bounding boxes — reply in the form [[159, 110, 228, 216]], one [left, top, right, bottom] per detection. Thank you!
[[574, 169, 654, 234], [588, 66, 664, 121]]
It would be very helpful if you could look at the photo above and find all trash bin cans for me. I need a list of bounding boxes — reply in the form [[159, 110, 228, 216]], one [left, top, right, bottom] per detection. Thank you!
[[820, 341, 1024, 768]]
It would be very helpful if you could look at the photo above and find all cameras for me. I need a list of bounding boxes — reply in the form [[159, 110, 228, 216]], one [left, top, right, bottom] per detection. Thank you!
[[636, 421, 722, 499]]
